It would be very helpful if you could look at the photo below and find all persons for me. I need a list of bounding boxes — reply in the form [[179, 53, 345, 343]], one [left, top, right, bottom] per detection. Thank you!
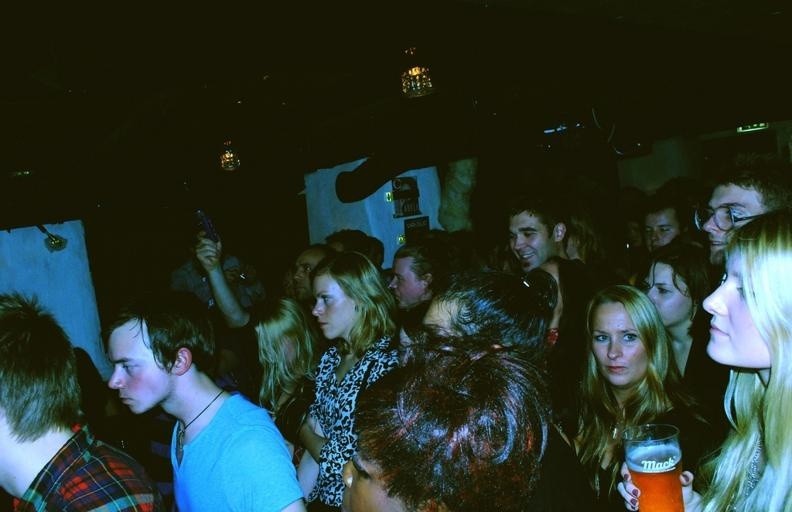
[[0, 76, 792, 512]]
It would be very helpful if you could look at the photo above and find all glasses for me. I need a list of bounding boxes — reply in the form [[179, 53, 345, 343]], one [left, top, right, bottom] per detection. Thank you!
[[693, 203, 762, 233]]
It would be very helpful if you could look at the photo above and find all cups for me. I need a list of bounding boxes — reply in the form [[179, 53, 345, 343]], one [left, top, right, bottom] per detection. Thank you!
[[619, 420, 686, 512]]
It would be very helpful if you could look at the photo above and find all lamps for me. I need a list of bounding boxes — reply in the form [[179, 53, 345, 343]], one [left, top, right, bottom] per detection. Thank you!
[[219, 135, 244, 173], [399, 45, 436, 97]]
